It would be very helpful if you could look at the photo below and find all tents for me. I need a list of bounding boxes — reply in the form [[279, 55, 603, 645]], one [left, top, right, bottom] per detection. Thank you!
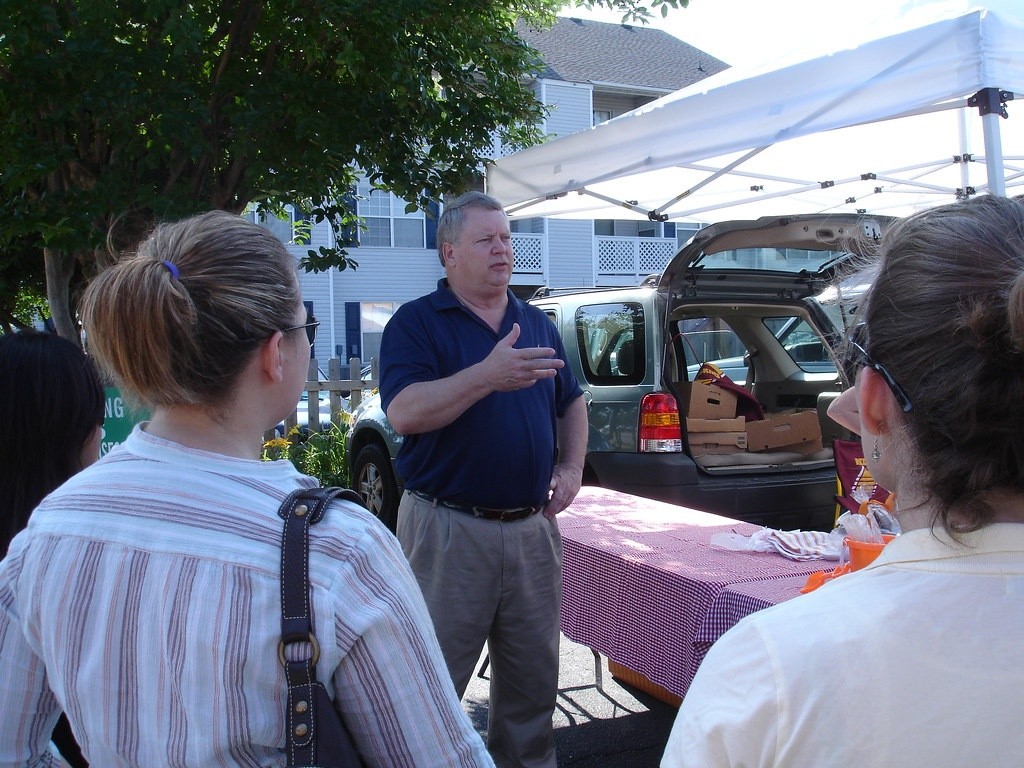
[[486, 1, 1024, 220]]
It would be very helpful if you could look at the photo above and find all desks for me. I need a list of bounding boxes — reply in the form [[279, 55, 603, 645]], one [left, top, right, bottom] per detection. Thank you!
[[479, 486, 845, 701]]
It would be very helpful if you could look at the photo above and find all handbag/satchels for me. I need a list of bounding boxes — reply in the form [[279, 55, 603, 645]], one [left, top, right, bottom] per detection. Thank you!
[[274, 486, 369, 768]]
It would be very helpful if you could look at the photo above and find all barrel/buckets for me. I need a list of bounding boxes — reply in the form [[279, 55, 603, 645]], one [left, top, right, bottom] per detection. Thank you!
[[842, 534, 896, 571]]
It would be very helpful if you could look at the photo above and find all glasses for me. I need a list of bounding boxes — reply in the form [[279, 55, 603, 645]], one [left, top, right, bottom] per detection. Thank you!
[[250, 316, 320, 347], [843, 321, 912, 412]]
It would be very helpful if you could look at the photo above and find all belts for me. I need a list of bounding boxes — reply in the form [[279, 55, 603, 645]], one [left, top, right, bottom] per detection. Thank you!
[[408, 488, 548, 523]]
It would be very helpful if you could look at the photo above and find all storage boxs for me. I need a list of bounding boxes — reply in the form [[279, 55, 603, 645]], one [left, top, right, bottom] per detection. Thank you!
[[670, 381, 824, 457]]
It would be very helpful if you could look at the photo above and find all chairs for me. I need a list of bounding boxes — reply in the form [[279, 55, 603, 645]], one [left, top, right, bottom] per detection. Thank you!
[[832, 439, 891, 528], [617, 340, 634, 377]]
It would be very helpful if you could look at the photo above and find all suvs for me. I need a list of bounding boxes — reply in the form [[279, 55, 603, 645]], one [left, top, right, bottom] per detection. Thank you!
[[343, 212, 902, 538]]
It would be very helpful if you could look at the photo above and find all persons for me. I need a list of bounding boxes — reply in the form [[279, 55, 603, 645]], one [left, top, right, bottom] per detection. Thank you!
[[0, 206, 498, 768], [375, 190, 591, 768], [659, 194, 1024, 768], [0, 331, 107, 767]]
